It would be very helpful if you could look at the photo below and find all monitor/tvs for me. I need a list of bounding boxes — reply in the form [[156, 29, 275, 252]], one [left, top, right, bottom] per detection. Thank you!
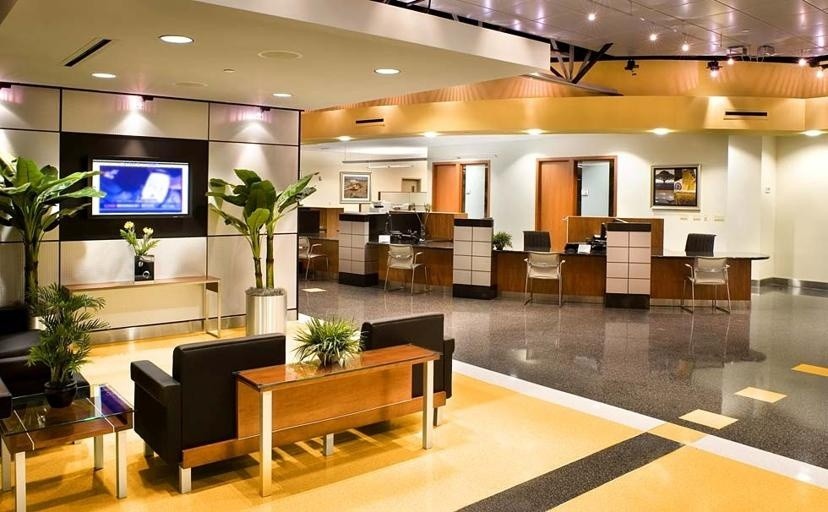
[[89, 159, 192, 220], [298, 210, 320, 233], [391, 212, 423, 238]]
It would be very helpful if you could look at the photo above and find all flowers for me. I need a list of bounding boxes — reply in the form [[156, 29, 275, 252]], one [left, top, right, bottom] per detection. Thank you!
[[119, 220, 158, 257]]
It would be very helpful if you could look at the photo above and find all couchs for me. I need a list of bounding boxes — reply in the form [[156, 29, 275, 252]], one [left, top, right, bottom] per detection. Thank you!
[[110, 310, 456, 494], [0, 297, 96, 422]]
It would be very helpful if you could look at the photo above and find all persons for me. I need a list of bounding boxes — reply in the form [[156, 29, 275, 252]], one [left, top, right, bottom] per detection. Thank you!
[[345, 179, 361, 190]]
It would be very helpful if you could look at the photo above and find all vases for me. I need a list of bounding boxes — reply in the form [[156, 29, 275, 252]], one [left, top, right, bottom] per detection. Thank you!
[[132, 256, 156, 282]]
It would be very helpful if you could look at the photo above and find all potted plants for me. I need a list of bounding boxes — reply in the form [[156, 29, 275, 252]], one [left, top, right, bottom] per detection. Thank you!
[[491, 232, 514, 252], [0, 154, 106, 332], [288, 311, 369, 369], [201, 165, 325, 341], [23, 280, 107, 408]]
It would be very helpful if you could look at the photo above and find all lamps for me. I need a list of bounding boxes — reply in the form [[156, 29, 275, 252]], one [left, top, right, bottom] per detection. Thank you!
[[706, 55, 725, 75], [801, 52, 827, 76], [365, 157, 412, 170], [623, 53, 638, 75]]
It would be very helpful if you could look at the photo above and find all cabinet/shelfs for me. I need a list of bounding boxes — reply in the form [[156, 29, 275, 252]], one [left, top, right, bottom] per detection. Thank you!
[[300, 204, 346, 235], [415, 209, 469, 242], [562, 215, 666, 255]]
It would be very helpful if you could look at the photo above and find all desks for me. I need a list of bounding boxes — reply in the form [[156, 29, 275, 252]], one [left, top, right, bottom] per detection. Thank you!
[[303, 233, 341, 281], [648, 248, 775, 310], [0, 381, 142, 512], [366, 238, 455, 287], [230, 340, 444, 497], [61, 274, 227, 341], [493, 246, 608, 305]]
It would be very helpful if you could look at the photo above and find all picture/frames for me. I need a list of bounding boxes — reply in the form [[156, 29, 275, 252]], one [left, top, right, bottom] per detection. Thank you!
[[339, 169, 372, 205], [647, 163, 701, 211]]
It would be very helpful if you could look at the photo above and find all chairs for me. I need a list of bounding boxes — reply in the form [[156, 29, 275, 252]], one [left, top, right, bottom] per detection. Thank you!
[[681, 231, 718, 255], [522, 251, 568, 307], [522, 229, 554, 251], [383, 241, 431, 297], [387, 210, 423, 244], [681, 255, 736, 315], [294, 231, 330, 283]]
